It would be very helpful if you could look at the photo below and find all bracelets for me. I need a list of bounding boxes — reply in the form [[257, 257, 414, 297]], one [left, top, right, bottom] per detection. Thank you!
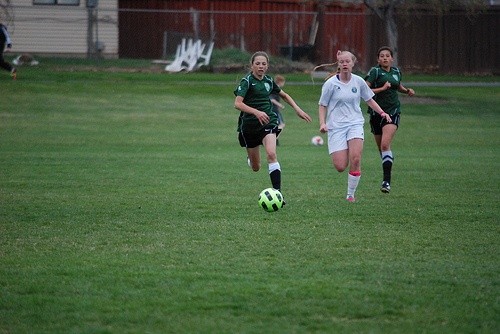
[[380, 112, 384, 115]]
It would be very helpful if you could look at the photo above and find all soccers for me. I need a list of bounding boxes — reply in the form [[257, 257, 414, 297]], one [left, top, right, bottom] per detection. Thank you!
[[311, 136, 325, 146], [258, 187, 283, 212]]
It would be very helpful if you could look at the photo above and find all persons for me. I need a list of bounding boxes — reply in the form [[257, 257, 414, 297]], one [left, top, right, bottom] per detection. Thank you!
[[318, 51, 392, 202], [363, 47, 415, 192], [270, 75, 286, 146], [234, 51, 312, 208], [0, 22, 17, 80]]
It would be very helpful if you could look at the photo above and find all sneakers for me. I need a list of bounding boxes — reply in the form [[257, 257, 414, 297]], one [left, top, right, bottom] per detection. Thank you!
[[11, 68, 17, 80], [346, 194, 355, 203], [380, 181, 390, 193]]
